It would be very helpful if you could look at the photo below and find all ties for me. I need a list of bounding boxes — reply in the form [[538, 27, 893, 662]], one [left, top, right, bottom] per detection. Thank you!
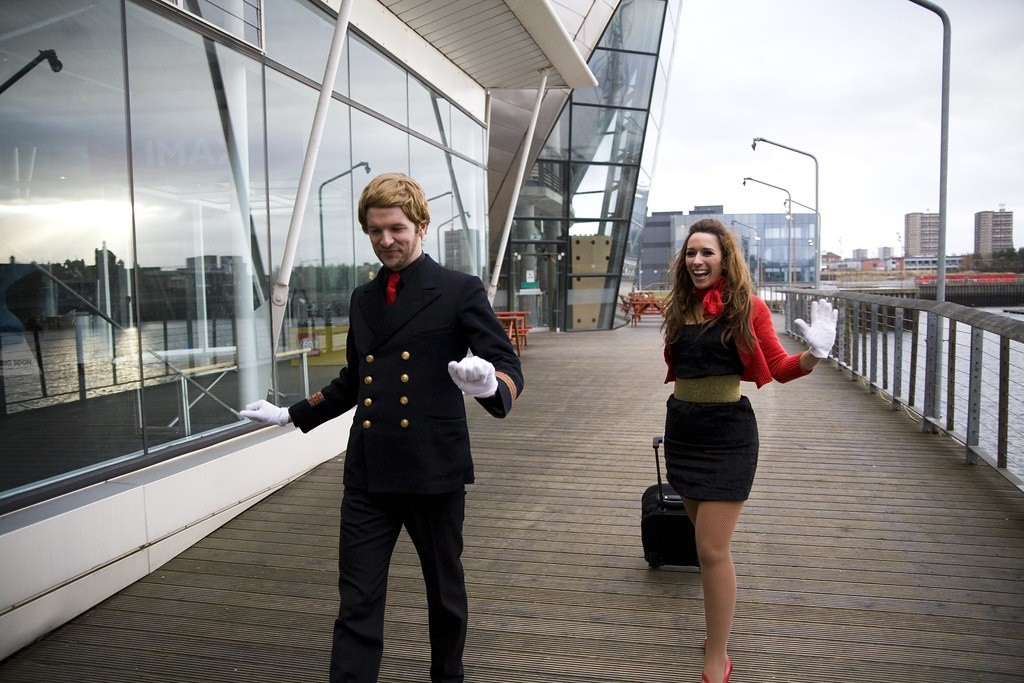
[[386, 273, 401, 309]]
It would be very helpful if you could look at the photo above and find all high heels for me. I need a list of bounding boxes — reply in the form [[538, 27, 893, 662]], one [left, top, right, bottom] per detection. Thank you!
[[702, 657, 733, 683]]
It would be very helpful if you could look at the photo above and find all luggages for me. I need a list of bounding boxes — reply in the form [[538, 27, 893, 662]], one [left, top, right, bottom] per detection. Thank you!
[[640, 438, 703, 570]]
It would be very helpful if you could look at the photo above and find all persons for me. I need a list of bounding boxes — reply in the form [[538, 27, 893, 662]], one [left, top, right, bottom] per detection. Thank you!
[[239, 172, 524, 683], [660, 218, 838, 683]]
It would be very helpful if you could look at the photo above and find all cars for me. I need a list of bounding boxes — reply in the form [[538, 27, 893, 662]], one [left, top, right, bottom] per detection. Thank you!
[[634, 283, 673, 298], [820, 274, 838, 285], [305, 297, 347, 317]]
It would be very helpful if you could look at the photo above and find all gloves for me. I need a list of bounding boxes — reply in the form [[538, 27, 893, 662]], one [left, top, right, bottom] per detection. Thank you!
[[239, 399, 287, 427], [794, 299, 838, 358], [448, 356, 498, 399]]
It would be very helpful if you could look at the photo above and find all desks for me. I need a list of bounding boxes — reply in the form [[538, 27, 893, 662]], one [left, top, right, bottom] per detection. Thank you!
[[617, 291, 665, 327], [494, 312, 531, 350], [497, 316, 521, 357], [503, 326, 532, 346]]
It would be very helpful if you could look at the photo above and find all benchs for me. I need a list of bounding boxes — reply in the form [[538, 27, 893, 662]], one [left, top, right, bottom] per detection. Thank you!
[[505, 329, 529, 337]]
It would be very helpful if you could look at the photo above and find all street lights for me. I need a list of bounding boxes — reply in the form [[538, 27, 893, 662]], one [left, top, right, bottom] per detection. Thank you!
[[751, 138, 819, 289], [897, 232, 903, 288], [731, 219, 762, 286], [318, 162, 371, 353], [743, 178, 791, 288], [784, 199, 821, 282], [437, 212, 471, 265]]
